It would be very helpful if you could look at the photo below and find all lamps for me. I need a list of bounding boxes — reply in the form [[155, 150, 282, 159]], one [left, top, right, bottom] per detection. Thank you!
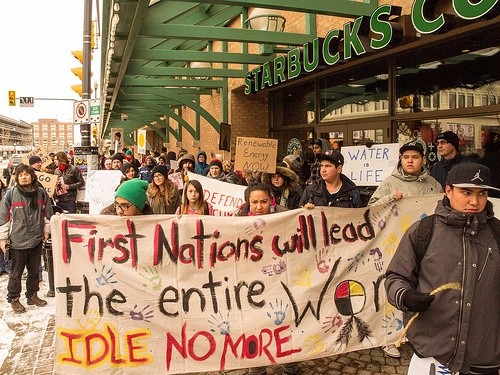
[[192, 61, 220, 93], [245, 7, 295, 56]]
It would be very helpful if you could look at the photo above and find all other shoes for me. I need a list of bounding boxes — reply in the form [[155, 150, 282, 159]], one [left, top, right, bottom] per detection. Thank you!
[[26, 291, 47, 307], [12, 296, 26, 314], [382, 344, 401, 357]]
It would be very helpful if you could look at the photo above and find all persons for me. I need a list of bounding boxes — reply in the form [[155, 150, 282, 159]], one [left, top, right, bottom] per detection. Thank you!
[[298, 150, 361, 209], [195, 138, 332, 209], [0, 165, 55, 314], [368, 141, 445, 358], [146, 165, 182, 214], [235, 182, 314, 375], [175, 179, 214, 216], [101, 148, 209, 183], [430, 131, 468, 191], [99, 179, 182, 220], [385, 164, 500, 375], [0, 150, 85, 276]]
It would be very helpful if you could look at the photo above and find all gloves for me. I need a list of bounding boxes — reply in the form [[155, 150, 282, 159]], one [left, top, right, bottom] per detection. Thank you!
[[400, 289, 434, 312]]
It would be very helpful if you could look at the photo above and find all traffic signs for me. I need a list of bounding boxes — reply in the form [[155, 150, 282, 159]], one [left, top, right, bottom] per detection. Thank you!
[[74, 99, 101, 125]]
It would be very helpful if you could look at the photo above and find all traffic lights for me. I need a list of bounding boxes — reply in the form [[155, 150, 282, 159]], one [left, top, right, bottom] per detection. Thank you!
[[8, 90, 16, 106], [70, 49, 93, 96]]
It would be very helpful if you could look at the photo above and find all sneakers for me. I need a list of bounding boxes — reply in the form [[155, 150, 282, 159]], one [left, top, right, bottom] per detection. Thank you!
[[21, 271, 42, 282]]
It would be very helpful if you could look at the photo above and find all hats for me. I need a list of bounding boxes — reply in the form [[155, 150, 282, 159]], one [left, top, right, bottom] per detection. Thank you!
[[399, 141, 424, 157], [151, 165, 168, 178], [112, 153, 124, 165], [115, 178, 148, 211], [316, 149, 344, 165], [179, 153, 195, 171], [309, 139, 322, 148], [283, 155, 302, 170], [445, 163, 500, 191], [29, 156, 42, 165], [436, 131, 459, 151], [125, 150, 131, 154], [209, 159, 223, 172]]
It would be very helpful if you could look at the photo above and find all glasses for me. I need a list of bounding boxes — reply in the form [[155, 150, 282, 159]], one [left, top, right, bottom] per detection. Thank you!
[[435, 140, 450, 146], [113, 203, 134, 210]]
